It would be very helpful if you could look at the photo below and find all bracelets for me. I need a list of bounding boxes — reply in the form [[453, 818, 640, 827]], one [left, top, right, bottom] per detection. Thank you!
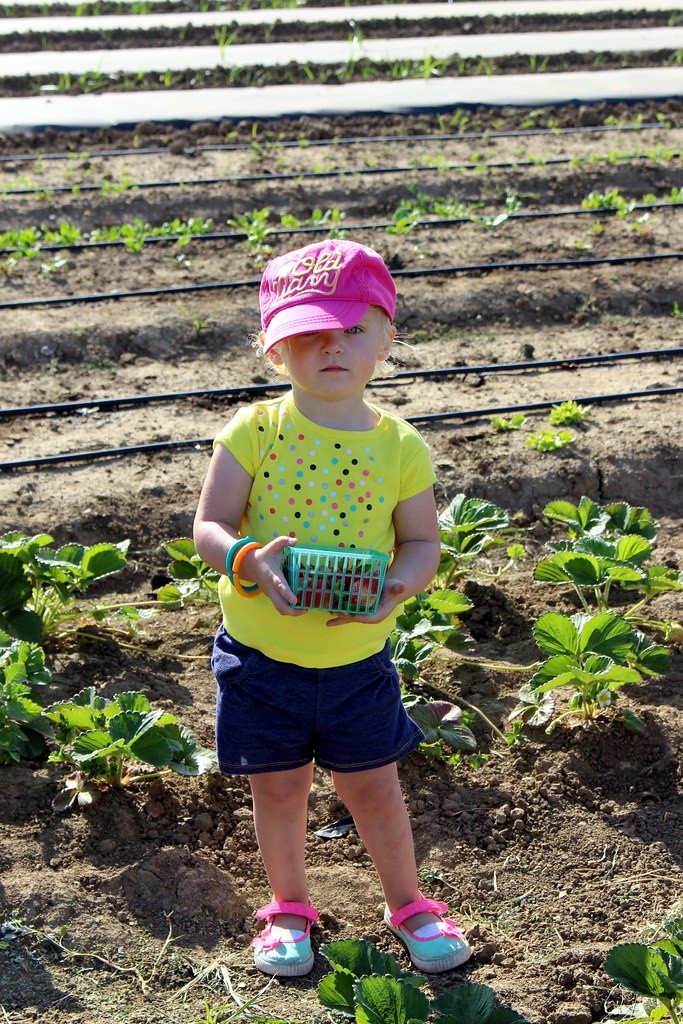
[[226, 537, 263, 598]]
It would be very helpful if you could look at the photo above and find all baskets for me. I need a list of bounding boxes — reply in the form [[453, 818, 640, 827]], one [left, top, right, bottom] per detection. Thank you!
[[281, 545, 389, 616]]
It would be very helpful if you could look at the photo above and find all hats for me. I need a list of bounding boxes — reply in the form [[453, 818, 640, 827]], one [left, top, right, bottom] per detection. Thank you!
[[259, 240, 396, 354]]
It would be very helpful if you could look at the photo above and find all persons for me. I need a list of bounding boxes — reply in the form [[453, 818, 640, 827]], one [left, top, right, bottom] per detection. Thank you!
[[192, 241, 472, 974]]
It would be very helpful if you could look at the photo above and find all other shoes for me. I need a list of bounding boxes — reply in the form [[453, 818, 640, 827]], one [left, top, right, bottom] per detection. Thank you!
[[252, 895, 319, 977], [383, 890, 471, 972]]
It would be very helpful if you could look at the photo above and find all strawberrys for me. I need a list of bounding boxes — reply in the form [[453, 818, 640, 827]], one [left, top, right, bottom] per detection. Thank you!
[[283, 556, 380, 609]]
